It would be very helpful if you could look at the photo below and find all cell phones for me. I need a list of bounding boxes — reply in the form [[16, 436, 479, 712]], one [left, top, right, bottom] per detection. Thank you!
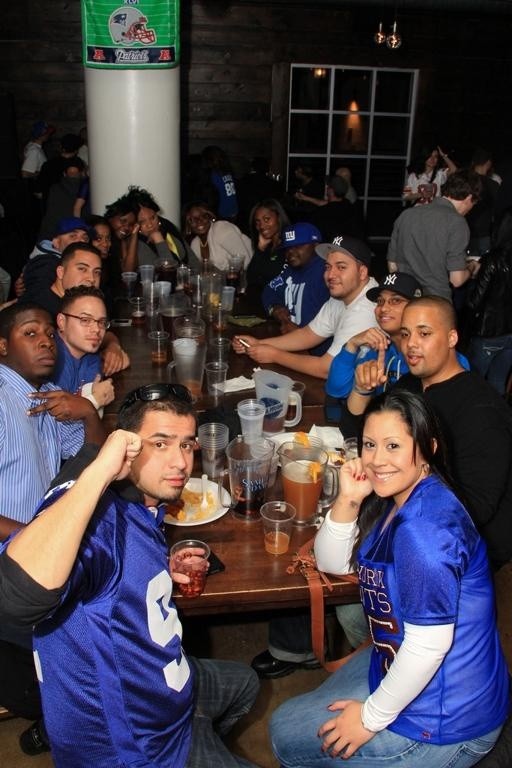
[[109, 319, 132, 327], [182, 534, 224, 576], [238, 338, 251, 348]]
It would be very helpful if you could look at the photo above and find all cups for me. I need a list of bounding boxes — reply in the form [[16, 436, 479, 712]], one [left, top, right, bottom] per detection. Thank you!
[[288, 379, 305, 399], [168, 538, 211, 596], [259, 500, 296, 555], [197, 421, 229, 478], [342, 436, 360, 457], [207, 361, 228, 397], [209, 336, 232, 361], [122, 262, 162, 323], [236, 397, 267, 442], [147, 330, 170, 365], [161, 254, 248, 330]]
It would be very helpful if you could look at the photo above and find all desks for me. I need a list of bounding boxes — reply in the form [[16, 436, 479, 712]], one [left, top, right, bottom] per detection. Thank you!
[[72, 287, 362, 621]]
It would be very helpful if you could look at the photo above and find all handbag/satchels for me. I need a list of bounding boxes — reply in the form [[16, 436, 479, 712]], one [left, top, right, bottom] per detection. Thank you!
[[287, 537, 359, 585]]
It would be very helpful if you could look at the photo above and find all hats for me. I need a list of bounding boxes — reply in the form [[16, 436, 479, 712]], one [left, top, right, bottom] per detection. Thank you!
[[315, 236, 371, 266], [57, 217, 89, 233], [281, 223, 321, 247], [366, 272, 422, 300]]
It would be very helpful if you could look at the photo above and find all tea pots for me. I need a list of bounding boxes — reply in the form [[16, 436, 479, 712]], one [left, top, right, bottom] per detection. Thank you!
[[251, 368, 303, 433], [172, 315, 207, 341], [218, 433, 273, 522], [277, 439, 338, 527], [165, 337, 206, 399]]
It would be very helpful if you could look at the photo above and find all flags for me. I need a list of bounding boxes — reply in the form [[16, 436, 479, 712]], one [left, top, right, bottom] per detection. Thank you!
[[81, 13, 180, 69]]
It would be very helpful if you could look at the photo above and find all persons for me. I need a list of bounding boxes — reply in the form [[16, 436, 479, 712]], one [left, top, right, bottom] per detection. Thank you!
[[266, 387, 511, 766], [0, 379, 263, 767], [186, 147, 511, 679], [1, 132, 187, 752]]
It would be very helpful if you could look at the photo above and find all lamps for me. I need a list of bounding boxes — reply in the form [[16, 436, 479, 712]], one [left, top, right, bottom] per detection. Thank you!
[[374, 22, 403, 49]]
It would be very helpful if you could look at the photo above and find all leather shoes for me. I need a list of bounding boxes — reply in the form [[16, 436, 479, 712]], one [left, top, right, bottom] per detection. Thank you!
[[253, 651, 324, 678]]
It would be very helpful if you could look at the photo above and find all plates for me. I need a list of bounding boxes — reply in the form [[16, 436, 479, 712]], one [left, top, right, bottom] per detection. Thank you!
[[155, 474, 231, 525], [269, 430, 311, 466]]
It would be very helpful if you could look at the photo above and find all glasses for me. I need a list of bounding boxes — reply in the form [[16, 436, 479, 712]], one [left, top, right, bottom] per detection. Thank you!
[[63, 313, 110, 329], [118, 383, 192, 409]]
